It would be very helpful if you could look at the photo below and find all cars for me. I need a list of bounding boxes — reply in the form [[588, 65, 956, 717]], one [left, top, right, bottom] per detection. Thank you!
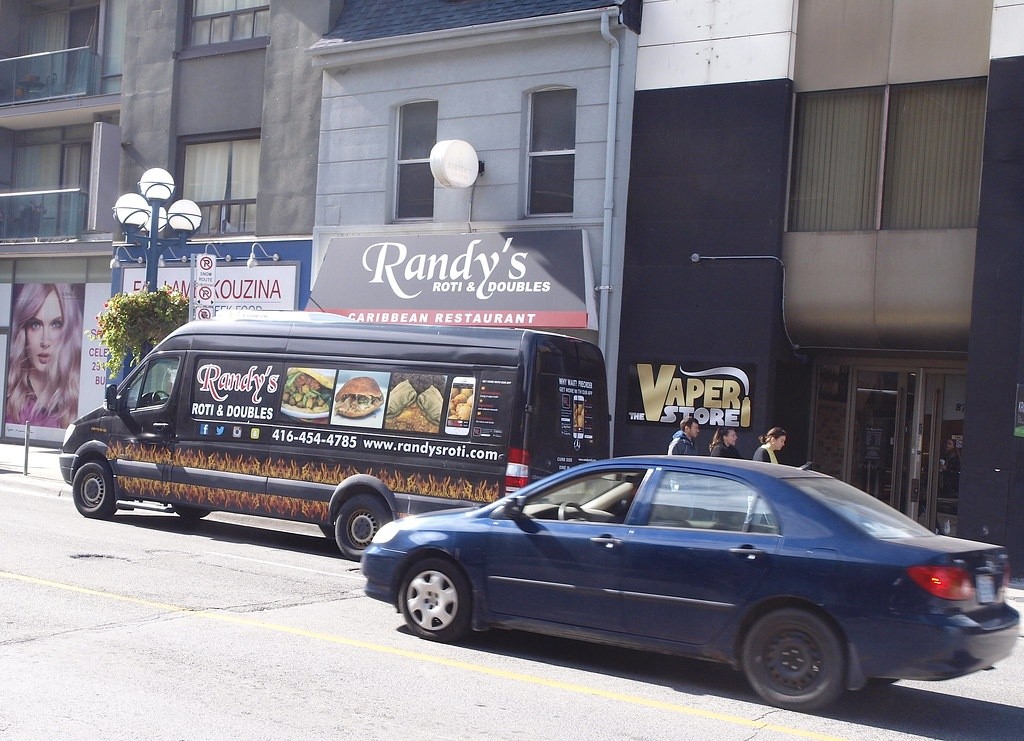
[[362, 455, 1021, 713]]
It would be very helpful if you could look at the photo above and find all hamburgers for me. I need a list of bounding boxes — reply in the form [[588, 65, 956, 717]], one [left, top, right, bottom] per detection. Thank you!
[[335, 377, 384, 417]]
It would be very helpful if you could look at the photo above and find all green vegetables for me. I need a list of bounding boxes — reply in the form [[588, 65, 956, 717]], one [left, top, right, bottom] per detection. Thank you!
[[285, 372, 332, 408]]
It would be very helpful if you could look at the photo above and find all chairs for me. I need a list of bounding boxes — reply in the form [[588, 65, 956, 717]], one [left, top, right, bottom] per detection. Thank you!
[[654, 492, 692, 528]]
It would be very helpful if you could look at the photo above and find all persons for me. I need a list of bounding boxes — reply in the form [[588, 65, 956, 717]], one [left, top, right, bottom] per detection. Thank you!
[[5, 283, 84, 430], [708, 426, 742, 459], [927, 438, 961, 497], [667, 417, 702, 466], [607, 485, 645, 525], [876, 449, 909, 490], [752, 426, 787, 466]]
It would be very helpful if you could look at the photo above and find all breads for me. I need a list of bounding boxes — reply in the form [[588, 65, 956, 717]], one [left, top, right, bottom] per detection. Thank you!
[[450, 387, 475, 421]]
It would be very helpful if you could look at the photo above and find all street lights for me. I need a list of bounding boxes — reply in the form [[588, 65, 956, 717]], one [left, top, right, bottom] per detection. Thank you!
[[111, 168, 203, 397]]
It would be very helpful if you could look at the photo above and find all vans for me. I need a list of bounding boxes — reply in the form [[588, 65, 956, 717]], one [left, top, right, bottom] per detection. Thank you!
[[59, 311, 611, 562]]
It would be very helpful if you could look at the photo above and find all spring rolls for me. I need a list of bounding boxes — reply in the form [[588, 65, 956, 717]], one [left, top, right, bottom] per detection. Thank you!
[[388, 380, 443, 426]]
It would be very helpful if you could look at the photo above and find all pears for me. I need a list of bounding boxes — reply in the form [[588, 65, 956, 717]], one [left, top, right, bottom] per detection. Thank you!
[[573, 404, 584, 426]]
